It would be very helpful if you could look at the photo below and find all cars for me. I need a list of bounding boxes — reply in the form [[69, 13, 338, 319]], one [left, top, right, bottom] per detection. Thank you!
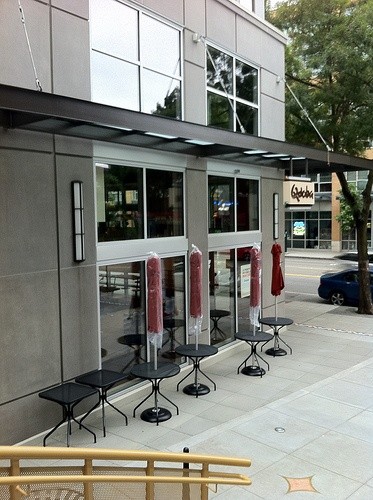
[[318, 266, 373, 306]]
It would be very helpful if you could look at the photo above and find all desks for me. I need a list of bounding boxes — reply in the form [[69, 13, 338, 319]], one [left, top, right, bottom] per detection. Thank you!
[[130, 361, 181, 426], [173, 343, 219, 398], [117, 334, 147, 373], [234, 331, 274, 378], [38, 382, 98, 448], [157, 319, 184, 357], [259, 317, 293, 358], [210, 309, 231, 341], [75, 368, 129, 437]]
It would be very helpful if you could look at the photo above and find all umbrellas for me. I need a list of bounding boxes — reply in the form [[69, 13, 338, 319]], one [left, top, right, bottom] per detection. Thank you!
[[146, 255, 164, 370], [270, 241, 285, 321], [250, 246, 261, 336], [189, 249, 203, 350]]
